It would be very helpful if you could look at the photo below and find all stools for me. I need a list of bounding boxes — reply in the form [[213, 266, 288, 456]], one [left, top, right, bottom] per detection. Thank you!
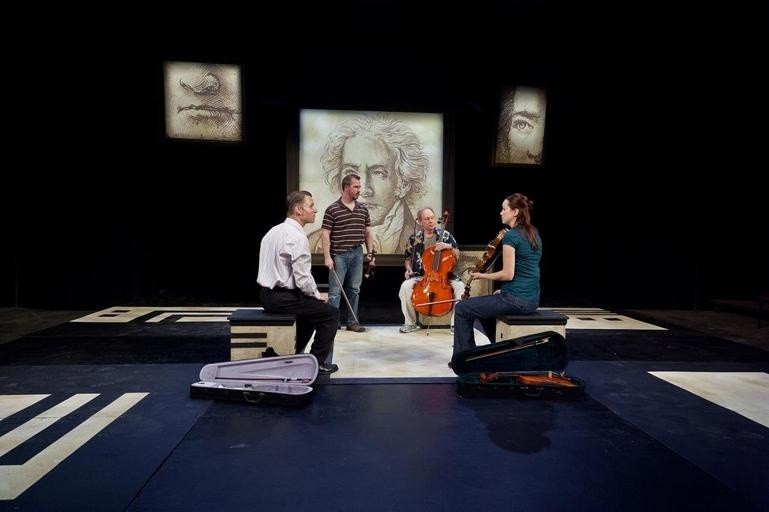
[[227, 309, 297, 362], [496, 310, 569, 343]]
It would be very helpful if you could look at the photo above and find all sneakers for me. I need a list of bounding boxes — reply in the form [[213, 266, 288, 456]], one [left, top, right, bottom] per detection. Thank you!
[[348, 322, 366, 332], [400, 324, 417, 333]]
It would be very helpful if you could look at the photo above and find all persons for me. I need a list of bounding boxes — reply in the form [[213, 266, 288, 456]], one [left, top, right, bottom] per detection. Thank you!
[[162, 62, 242, 140], [322, 173, 374, 332], [257, 190, 340, 372], [305, 110, 439, 254], [498, 86, 546, 163], [447, 193, 542, 368], [397, 208, 465, 333]]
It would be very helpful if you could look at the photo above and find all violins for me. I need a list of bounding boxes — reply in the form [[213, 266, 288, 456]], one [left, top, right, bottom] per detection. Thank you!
[[480, 370, 576, 388], [462, 229, 507, 299]]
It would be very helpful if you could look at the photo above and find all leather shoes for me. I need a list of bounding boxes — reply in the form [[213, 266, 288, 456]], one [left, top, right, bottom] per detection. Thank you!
[[319, 362, 338, 373]]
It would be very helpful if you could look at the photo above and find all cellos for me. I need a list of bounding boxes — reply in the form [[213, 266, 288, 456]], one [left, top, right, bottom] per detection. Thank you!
[[412, 210, 455, 317]]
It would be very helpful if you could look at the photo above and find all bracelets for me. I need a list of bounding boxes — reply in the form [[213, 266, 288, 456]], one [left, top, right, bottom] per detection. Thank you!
[[368, 252, 371, 255]]
